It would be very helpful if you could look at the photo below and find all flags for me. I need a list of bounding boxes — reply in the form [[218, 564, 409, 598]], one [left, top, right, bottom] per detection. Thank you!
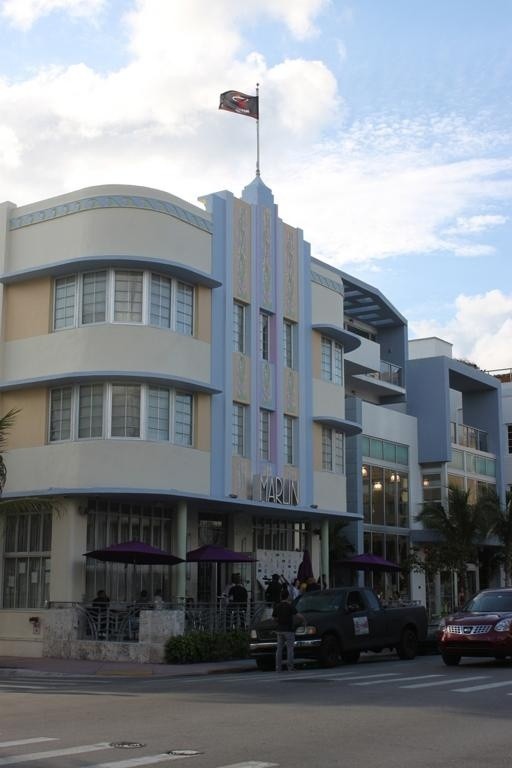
[[220, 91, 259, 120]]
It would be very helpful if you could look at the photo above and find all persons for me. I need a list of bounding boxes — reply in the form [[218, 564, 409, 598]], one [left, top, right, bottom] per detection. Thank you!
[[457, 585, 464, 607], [94, 587, 110, 609], [138, 589, 148, 605], [265, 573, 281, 601], [306, 577, 323, 593], [288, 578, 304, 599], [153, 588, 163, 605], [223, 573, 247, 626], [270, 590, 308, 674], [393, 591, 404, 604]]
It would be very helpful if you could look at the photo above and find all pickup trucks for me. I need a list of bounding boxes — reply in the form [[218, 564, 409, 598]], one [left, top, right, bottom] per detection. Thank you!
[[240, 581, 431, 673]]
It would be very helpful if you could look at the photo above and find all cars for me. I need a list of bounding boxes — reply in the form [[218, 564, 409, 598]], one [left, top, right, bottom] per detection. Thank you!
[[439, 588, 511, 668]]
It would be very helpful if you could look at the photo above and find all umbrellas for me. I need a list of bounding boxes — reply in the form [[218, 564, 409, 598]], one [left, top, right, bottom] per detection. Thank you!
[[82, 538, 186, 598], [296, 549, 314, 582], [343, 552, 403, 590], [187, 544, 260, 590]]
